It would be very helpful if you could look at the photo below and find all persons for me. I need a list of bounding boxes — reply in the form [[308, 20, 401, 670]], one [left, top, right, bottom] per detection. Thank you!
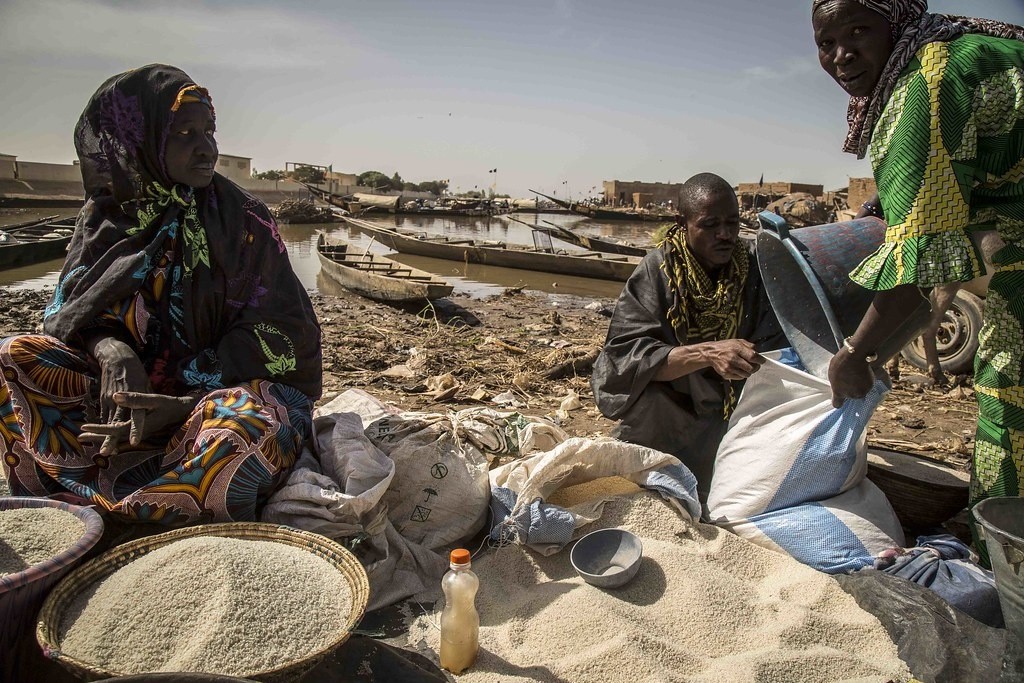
[[0, 64, 324, 534], [812, 0, 1023, 569], [590, 174, 793, 508]]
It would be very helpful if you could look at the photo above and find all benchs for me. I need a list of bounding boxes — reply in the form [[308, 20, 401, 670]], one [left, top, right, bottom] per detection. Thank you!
[[317, 232, 653, 284], [0, 214, 82, 244]]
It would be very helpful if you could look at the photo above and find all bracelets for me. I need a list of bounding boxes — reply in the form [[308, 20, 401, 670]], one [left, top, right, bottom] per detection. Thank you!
[[843, 336, 878, 363], [861, 201, 884, 221]]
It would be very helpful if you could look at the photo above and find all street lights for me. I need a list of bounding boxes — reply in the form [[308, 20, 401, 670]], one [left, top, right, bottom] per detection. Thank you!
[[563, 181, 568, 204], [489, 168, 498, 199]]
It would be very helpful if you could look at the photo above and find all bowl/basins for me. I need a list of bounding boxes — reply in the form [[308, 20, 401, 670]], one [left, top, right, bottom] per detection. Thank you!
[[570, 528, 643, 589]]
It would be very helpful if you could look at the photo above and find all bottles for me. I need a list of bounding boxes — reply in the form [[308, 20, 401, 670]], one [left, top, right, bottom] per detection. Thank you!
[[439, 549, 480, 674]]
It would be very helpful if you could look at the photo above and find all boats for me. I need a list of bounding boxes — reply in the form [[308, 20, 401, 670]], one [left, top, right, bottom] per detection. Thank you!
[[504, 215, 650, 257], [529, 188, 681, 222], [0, 215, 79, 269], [315, 231, 455, 302], [331, 212, 640, 283]]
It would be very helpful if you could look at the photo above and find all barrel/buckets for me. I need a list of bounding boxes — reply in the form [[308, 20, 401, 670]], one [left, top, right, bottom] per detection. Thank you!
[[972, 494, 1024, 657], [755, 209, 937, 384]]
